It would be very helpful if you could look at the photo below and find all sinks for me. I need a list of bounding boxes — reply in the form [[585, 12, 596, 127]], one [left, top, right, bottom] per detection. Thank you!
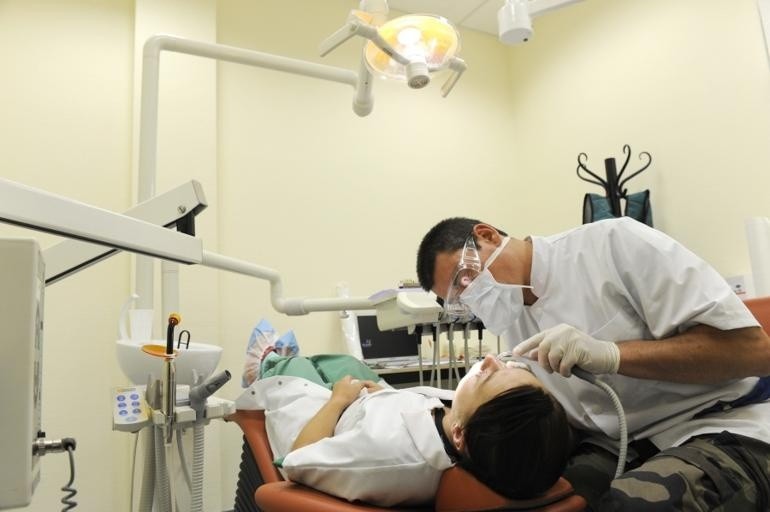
[[114, 337, 224, 390]]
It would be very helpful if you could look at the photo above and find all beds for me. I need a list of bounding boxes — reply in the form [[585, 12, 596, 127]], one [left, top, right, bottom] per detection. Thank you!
[[220, 404, 587, 512]]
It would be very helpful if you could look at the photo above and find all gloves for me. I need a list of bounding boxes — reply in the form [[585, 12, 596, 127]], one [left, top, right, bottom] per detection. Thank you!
[[514, 323, 620, 378]]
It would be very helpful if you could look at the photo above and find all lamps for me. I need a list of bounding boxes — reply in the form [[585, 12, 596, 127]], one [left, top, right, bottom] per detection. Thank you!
[[363, 5, 462, 92]]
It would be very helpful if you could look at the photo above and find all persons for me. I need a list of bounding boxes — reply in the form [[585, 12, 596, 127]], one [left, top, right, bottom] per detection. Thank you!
[[415, 216, 770, 512], [234, 316, 573, 508]]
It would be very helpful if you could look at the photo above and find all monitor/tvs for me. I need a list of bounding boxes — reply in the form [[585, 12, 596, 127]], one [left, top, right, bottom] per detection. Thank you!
[[341, 310, 419, 366]]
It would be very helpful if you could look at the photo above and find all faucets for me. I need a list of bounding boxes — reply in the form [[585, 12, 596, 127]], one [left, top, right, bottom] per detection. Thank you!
[[116, 291, 141, 341]]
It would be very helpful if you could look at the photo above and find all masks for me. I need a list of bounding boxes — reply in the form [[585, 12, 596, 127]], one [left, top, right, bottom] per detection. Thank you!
[[459, 237, 524, 335]]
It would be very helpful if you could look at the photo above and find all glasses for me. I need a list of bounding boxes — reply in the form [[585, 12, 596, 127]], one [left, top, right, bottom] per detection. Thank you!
[[436, 235, 481, 319]]
